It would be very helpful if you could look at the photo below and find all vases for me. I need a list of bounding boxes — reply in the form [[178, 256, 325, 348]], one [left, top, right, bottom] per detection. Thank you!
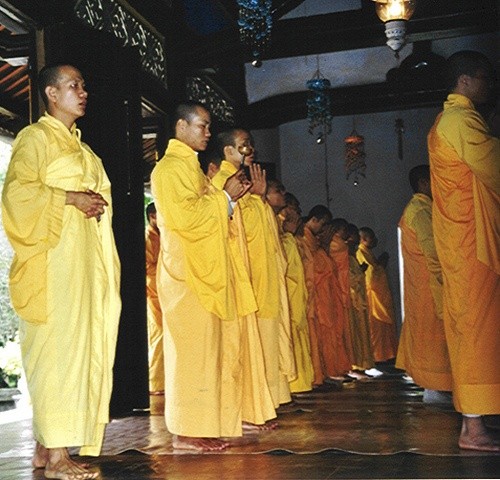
[[8, 374, 17, 387]]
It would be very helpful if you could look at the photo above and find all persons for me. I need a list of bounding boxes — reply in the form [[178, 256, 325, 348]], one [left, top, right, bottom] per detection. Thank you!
[[208, 126, 399, 431], [2, 63, 121, 480], [150, 102, 257, 451], [146, 204, 163, 399], [429, 51, 500, 449], [396, 164, 455, 390]]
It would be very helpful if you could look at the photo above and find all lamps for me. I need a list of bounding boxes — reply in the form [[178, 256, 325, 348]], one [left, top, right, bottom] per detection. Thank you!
[[373, 0, 419, 57]]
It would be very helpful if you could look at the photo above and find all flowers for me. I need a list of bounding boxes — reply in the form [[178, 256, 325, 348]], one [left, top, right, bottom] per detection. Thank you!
[[4, 360, 21, 377]]
